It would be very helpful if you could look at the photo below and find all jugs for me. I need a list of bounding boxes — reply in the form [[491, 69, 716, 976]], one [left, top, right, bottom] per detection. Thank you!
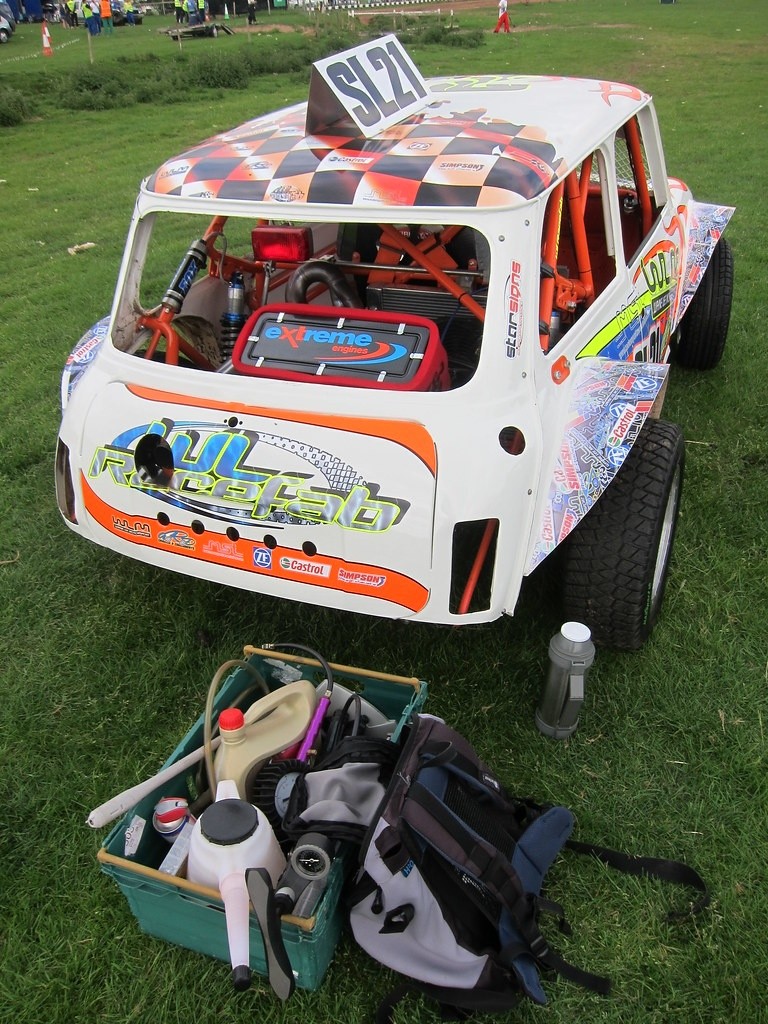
[[533, 619, 596, 740], [186, 778, 288, 993]]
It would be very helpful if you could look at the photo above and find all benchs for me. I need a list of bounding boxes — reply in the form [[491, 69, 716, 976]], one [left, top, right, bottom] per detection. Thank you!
[[554, 184, 640, 289]]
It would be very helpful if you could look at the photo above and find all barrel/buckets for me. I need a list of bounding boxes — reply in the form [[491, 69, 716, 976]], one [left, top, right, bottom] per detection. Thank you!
[[211, 679, 317, 864]]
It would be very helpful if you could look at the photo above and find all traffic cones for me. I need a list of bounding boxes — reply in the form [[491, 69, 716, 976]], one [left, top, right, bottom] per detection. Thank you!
[[42, 26, 52, 56], [223, 3, 230, 20], [41, 20, 53, 43]]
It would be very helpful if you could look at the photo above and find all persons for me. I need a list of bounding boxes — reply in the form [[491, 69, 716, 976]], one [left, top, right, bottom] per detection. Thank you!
[[494, 0, 512, 33], [174, 0, 209, 26], [248, 0, 257, 25], [0, 0, 136, 36]]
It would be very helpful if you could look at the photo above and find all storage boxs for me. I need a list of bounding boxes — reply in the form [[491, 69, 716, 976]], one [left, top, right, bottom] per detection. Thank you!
[[92, 652, 427, 996]]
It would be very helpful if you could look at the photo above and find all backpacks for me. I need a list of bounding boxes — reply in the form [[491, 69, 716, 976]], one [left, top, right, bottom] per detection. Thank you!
[[280, 709, 712, 1020]]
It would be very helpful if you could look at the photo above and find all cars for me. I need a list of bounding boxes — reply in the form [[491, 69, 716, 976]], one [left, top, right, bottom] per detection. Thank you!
[[0, 0, 145, 43], [50, 73, 738, 654]]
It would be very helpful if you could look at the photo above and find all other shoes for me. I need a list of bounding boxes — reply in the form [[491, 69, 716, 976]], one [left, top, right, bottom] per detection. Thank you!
[[493, 31, 498, 34], [70, 26, 75, 29], [249, 21, 258, 25]]
